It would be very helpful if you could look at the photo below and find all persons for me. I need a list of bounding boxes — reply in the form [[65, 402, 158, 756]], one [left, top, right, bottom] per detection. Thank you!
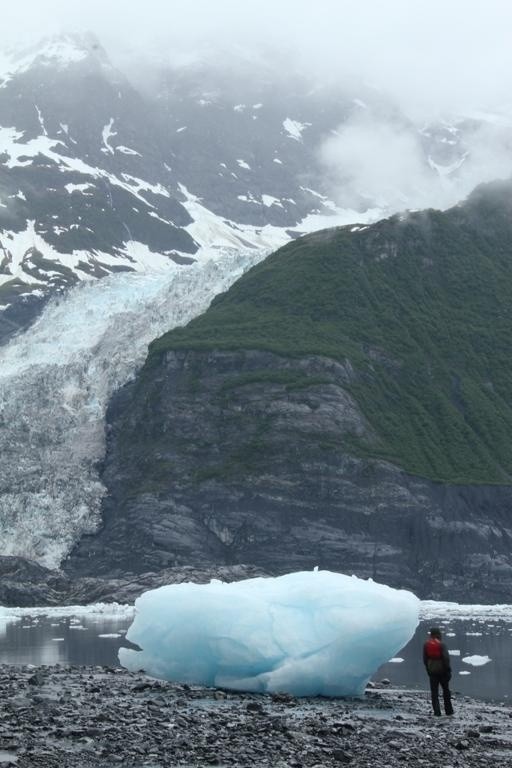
[[423, 627, 453, 716]]
